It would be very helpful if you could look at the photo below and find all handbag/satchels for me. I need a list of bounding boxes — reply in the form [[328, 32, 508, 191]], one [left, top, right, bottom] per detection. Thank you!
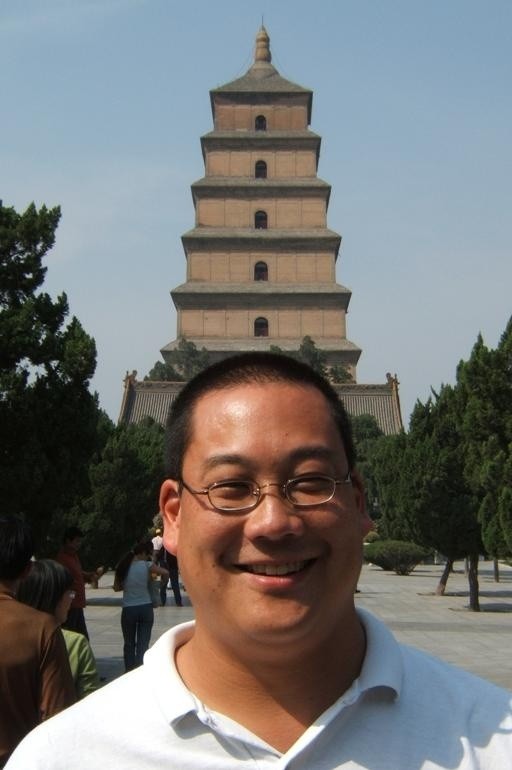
[[148, 581, 166, 606]]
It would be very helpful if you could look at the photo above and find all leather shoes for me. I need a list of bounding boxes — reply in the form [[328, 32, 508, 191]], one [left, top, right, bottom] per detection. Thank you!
[[160, 602, 166, 607], [176, 600, 182, 606]]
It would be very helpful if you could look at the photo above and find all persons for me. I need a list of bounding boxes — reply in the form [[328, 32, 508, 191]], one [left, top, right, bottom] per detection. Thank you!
[[2, 350, 512, 770], [1, 530, 101, 769], [113, 529, 183, 674]]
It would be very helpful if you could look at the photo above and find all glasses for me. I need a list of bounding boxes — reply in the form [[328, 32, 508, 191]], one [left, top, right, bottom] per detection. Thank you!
[[65, 589, 76, 599], [181, 472, 352, 516]]
